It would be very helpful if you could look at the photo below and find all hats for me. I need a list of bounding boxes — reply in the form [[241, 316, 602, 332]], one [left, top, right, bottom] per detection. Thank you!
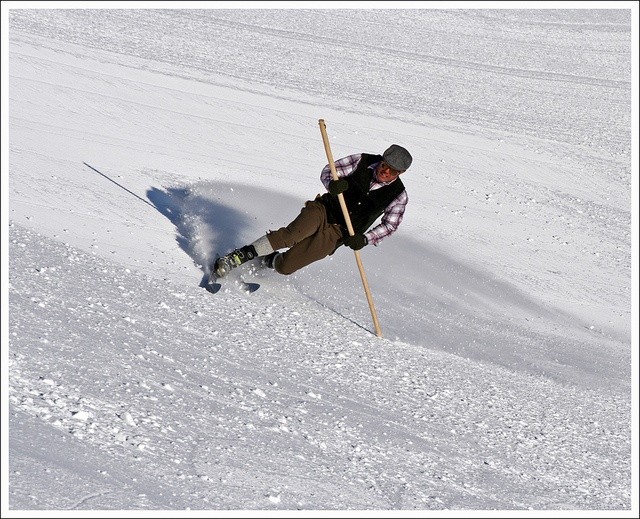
[[383, 144, 413, 172]]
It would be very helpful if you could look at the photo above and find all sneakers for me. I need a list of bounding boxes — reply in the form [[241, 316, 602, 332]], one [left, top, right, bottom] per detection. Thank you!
[[214, 250, 242, 277]]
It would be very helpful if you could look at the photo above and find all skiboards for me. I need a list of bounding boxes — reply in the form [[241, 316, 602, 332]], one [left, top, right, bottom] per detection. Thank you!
[[206, 253, 260, 294]]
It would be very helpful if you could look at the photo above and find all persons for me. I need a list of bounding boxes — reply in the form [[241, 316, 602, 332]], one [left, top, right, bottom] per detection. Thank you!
[[214, 143, 413, 281]]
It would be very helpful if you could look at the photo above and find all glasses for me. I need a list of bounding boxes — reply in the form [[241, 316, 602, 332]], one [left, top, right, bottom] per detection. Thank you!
[[382, 162, 398, 176]]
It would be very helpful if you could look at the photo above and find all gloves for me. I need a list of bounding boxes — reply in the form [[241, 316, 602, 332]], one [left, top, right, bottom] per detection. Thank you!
[[344, 233, 369, 250], [328, 178, 349, 195]]
